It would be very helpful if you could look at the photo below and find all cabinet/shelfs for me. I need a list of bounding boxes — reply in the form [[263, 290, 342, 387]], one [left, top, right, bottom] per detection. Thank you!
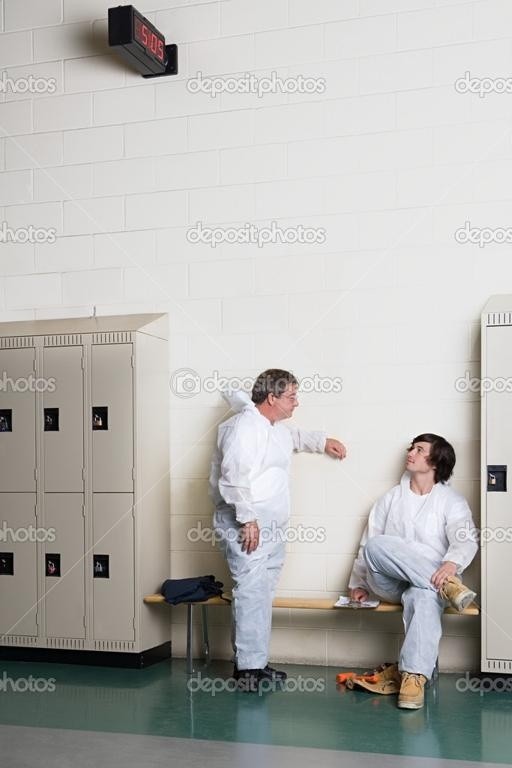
[[0, 312, 177, 661]]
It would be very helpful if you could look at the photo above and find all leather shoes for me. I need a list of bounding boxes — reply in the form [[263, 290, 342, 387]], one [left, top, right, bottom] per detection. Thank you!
[[255, 666, 287, 680], [233, 664, 259, 692]]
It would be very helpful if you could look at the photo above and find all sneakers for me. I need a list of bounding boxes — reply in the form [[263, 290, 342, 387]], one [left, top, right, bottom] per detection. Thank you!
[[397, 672, 427, 709], [441, 575, 477, 613]]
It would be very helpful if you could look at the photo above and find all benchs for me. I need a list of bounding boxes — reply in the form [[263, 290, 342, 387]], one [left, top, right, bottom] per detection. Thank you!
[[146, 571, 472, 698]]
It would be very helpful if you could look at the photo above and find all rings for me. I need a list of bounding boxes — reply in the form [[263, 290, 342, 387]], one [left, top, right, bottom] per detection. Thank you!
[[246, 539, 250, 542]]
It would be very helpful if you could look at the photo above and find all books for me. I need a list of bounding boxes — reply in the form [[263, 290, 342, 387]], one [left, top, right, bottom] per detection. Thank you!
[[333, 594, 381, 610]]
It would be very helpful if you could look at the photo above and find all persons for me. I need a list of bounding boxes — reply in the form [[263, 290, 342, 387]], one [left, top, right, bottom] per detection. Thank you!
[[206, 368, 346, 692], [395, 705, 443, 768], [230, 693, 279, 768], [345, 432, 480, 714]]
